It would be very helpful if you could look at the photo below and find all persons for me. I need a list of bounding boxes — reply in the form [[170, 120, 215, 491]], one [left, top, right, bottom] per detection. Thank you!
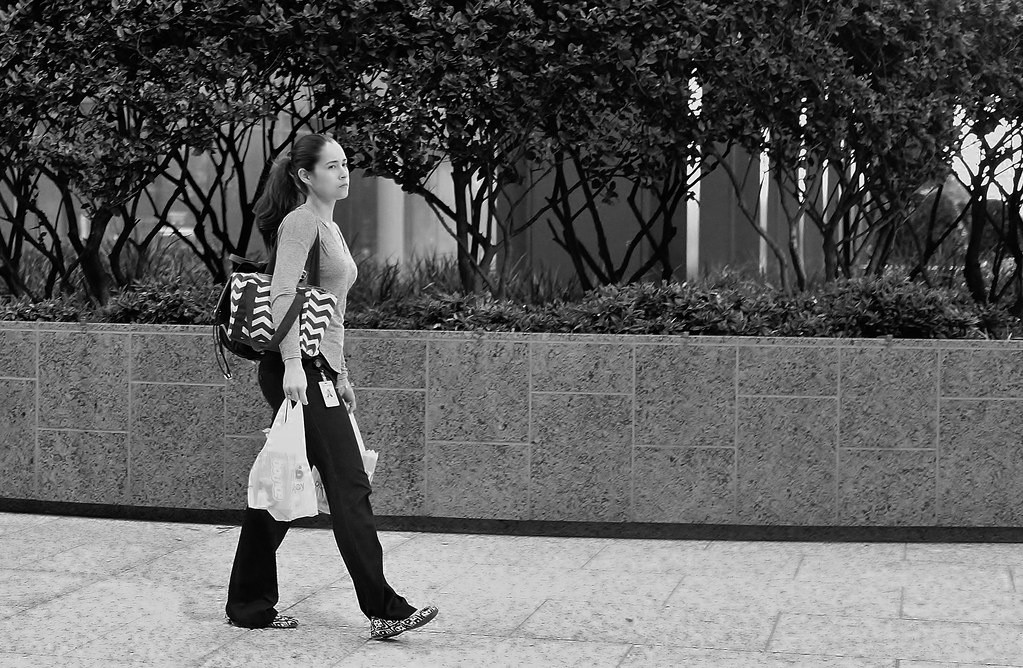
[[225, 134, 439, 640]]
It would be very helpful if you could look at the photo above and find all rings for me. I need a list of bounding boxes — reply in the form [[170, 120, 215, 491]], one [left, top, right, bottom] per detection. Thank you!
[[288, 394, 292, 395]]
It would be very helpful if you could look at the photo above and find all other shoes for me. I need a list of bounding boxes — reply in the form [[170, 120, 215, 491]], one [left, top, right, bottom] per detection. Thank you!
[[370, 605, 438, 640], [227, 614, 298, 628]]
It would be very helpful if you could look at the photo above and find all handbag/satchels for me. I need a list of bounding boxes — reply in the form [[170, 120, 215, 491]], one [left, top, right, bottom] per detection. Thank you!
[[247, 396, 319, 521], [211, 210, 339, 379], [311, 401, 379, 515]]
